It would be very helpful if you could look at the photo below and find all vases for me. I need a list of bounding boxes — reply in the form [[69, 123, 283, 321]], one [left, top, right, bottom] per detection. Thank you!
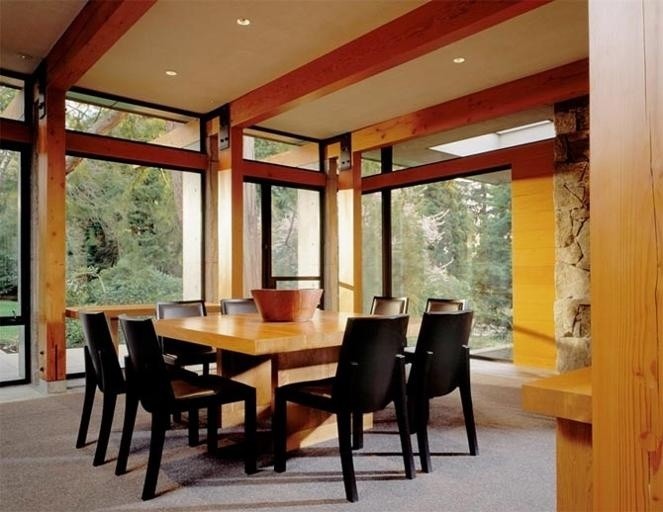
[[250, 289, 324, 322]]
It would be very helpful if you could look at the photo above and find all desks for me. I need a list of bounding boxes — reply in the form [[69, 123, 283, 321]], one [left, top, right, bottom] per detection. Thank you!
[[153, 312, 367, 453]]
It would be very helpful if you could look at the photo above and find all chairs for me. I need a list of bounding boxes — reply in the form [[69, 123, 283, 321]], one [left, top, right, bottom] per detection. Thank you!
[[407, 310, 479, 473], [370, 296, 409, 346], [274, 315, 416, 502], [76, 311, 198, 466], [405, 298, 465, 362], [115, 314, 256, 501], [221, 298, 257, 313], [157, 301, 217, 423]]
